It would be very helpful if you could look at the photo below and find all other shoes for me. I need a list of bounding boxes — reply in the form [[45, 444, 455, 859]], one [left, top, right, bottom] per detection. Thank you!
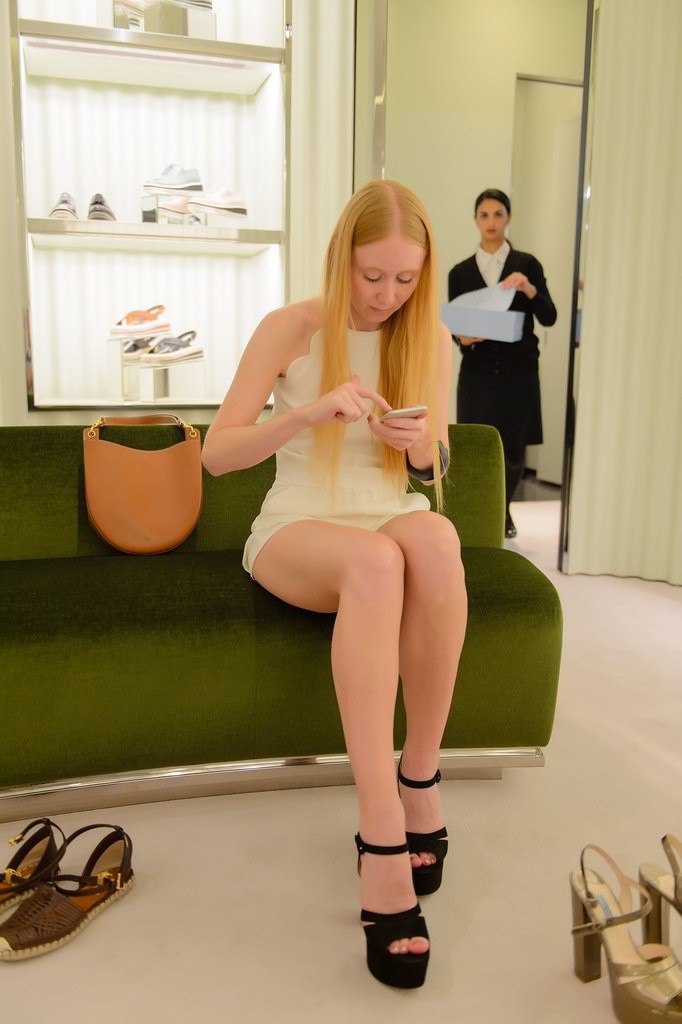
[[159, 192, 246, 218], [504, 500, 517, 538], [88, 193, 116, 220], [48, 192, 78, 220], [143, 162, 203, 192]]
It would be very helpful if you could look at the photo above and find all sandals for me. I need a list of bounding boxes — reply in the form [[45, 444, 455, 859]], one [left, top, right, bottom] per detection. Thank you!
[[109, 304, 170, 335], [0, 823, 135, 962], [141, 330, 202, 363], [122, 336, 167, 359], [0, 816, 66, 912]]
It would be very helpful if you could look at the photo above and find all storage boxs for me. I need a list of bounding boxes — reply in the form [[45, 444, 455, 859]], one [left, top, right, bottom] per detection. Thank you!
[[440, 301, 525, 342]]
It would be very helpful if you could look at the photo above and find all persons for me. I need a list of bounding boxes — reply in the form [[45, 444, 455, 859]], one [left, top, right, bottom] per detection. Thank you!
[[202, 181, 468, 990], [447, 188, 557, 537]]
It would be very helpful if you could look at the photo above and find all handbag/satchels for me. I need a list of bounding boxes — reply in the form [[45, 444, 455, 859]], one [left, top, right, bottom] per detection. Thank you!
[[83, 414, 203, 555]]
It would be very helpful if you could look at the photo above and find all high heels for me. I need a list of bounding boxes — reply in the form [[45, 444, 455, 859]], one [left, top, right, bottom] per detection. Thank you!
[[636, 832, 682, 959], [570, 844, 682, 1024], [353, 826, 431, 989], [396, 753, 449, 896]]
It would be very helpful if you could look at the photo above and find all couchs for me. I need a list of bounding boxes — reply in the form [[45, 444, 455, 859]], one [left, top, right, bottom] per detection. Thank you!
[[1, 423, 564, 822]]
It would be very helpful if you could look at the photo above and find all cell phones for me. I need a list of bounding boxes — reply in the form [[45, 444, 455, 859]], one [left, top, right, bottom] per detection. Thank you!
[[379, 407, 427, 424]]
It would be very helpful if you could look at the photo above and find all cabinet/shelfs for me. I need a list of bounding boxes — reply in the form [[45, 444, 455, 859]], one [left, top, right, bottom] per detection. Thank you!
[[1, 0, 293, 426]]
